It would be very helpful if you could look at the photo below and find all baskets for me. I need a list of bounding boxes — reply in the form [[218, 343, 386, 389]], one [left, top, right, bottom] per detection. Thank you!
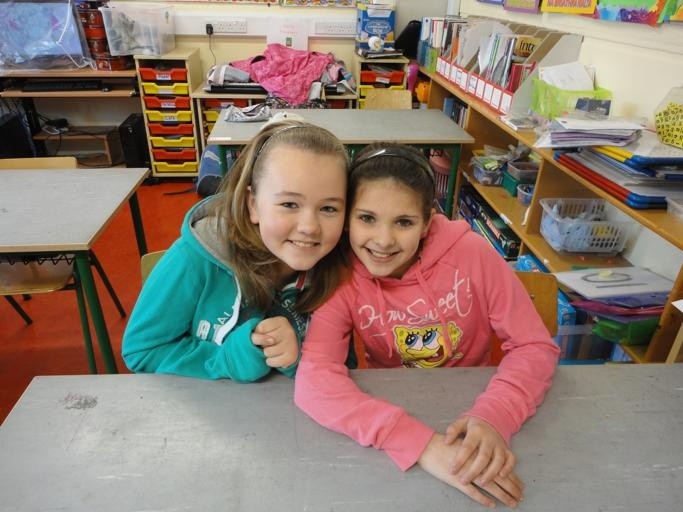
[[538, 197, 632, 258]]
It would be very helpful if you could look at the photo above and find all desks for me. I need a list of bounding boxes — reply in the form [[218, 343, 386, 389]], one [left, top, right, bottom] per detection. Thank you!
[[191, 82, 358, 149], [1, 66, 136, 157], [205, 108, 475, 218], [0, 167, 149, 375], [0, 363, 683, 512]]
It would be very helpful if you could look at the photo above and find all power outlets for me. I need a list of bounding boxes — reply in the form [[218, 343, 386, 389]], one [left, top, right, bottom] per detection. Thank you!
[[206, 21, 247, 33], [327, 21, 357, 35]]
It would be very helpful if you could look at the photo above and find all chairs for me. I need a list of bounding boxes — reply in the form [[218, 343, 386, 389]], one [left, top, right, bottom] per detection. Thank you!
[[140, 250, 165, 286], [0, 157, 126, 325]]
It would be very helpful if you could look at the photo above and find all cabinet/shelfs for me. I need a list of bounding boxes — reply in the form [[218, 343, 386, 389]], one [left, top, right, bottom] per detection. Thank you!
[[417, 65, 683, 363], [352, 53, 409, 109], [135, 48, 203, 178], [32, 125, 124, 167]]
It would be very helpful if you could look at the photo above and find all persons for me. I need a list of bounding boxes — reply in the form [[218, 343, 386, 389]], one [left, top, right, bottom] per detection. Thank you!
[[291, 139, 562, 510], [119, 113, 353, 380]]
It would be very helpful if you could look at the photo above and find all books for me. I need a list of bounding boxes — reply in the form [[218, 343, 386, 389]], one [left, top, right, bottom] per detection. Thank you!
[[419, 16, 543, 95], [455, 182, 520, 263], [498, 113, 536, 132]]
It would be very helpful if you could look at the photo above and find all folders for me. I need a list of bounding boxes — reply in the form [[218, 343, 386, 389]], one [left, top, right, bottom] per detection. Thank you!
[[417, 17, 584, 116]]
